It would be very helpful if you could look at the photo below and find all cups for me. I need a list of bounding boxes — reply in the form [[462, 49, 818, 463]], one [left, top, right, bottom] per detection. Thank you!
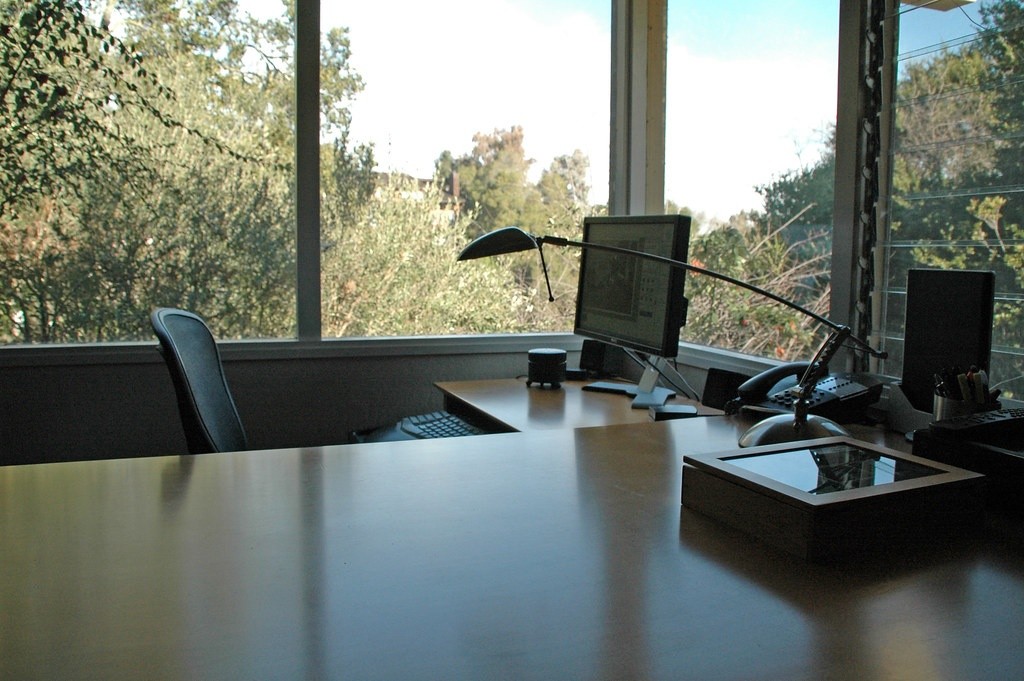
[[932, 393, 965, 422]]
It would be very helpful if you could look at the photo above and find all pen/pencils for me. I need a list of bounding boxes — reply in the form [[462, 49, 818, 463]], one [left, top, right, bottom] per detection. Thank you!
[[930, 358, 1002, 404]]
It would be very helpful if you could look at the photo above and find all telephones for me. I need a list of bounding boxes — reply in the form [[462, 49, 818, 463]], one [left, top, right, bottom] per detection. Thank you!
[[735, 364, 884, 423]]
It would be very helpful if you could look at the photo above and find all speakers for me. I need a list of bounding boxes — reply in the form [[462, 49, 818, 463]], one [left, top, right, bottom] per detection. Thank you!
[[579, 339, 624, 377], [701, 368, 766, 412]]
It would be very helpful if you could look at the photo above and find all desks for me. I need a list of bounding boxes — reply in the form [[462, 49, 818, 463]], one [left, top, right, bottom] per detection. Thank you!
[[0, 415, 1023, 681], [433, 377, 726, 433]]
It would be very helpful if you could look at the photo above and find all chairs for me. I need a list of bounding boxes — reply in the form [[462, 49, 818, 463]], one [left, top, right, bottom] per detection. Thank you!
[[149, 308, 249, 455]]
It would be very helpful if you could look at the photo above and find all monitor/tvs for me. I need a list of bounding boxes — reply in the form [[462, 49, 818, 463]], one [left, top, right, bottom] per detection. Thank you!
[[574, 214, 691, 410]]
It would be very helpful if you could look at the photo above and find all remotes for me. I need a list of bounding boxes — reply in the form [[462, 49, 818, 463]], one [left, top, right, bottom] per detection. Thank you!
[[927, 407, 1024, 439]]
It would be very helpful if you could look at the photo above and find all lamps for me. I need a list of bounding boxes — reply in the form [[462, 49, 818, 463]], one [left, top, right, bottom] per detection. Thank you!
[[457, 226, 889, 449]]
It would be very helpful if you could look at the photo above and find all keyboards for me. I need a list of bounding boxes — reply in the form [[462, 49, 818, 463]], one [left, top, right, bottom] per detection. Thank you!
[[363, 410, 488, 446]]
